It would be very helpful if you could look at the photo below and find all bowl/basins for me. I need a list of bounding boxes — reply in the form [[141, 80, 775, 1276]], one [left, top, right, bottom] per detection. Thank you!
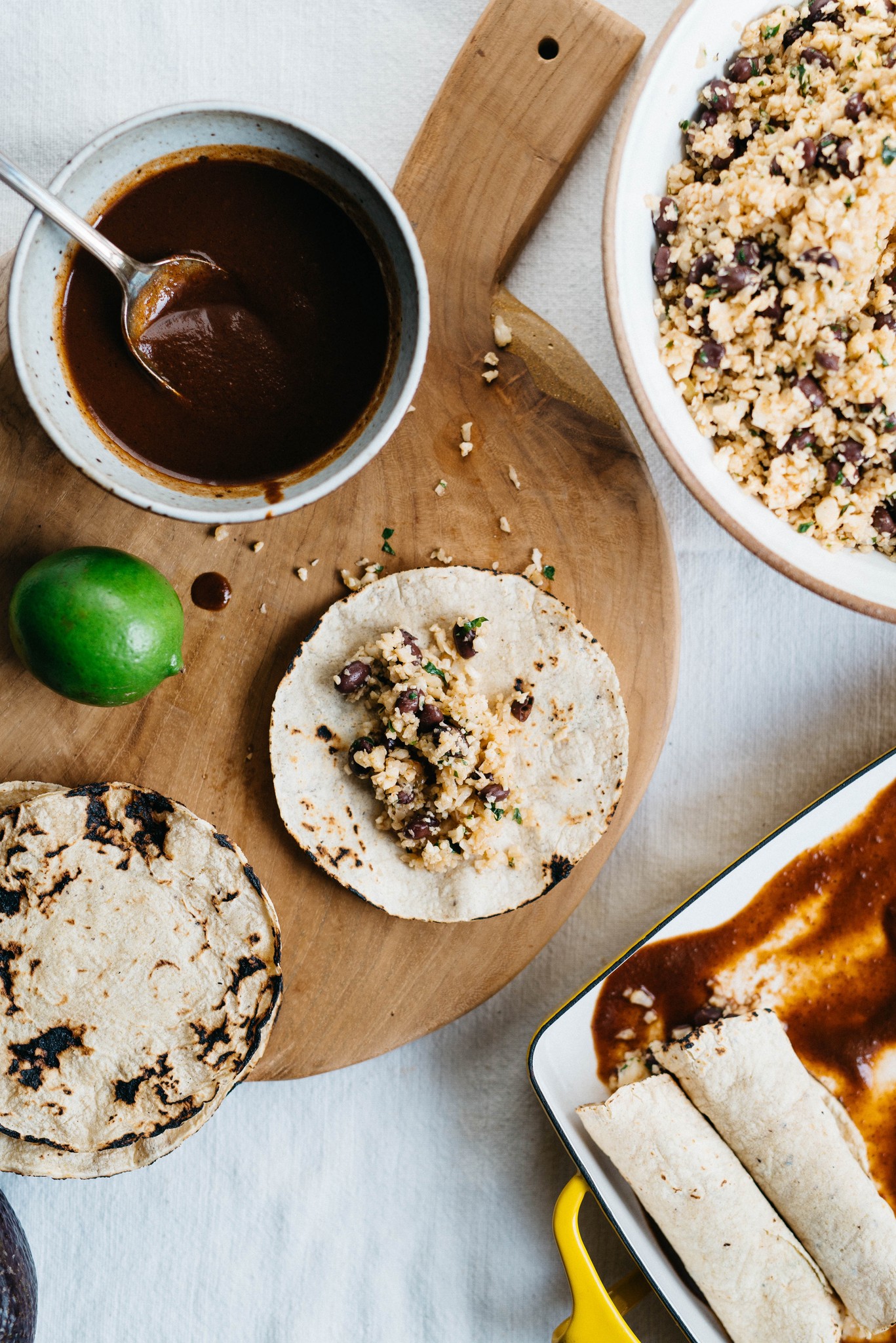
[[3, 104, 433, 525]]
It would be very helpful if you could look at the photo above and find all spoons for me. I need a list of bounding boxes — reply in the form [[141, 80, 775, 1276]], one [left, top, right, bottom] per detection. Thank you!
[[0, 148, 256, 403]]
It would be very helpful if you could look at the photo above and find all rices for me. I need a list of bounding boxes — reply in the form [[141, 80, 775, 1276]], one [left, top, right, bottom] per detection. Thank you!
[[332, 627, 547, 876], [645, 0, 896, 567]]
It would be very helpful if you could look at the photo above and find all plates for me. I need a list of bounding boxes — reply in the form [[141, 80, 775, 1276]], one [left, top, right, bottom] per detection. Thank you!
[[594, 1, 895, 649], [522, 728, 896, 1343]]
